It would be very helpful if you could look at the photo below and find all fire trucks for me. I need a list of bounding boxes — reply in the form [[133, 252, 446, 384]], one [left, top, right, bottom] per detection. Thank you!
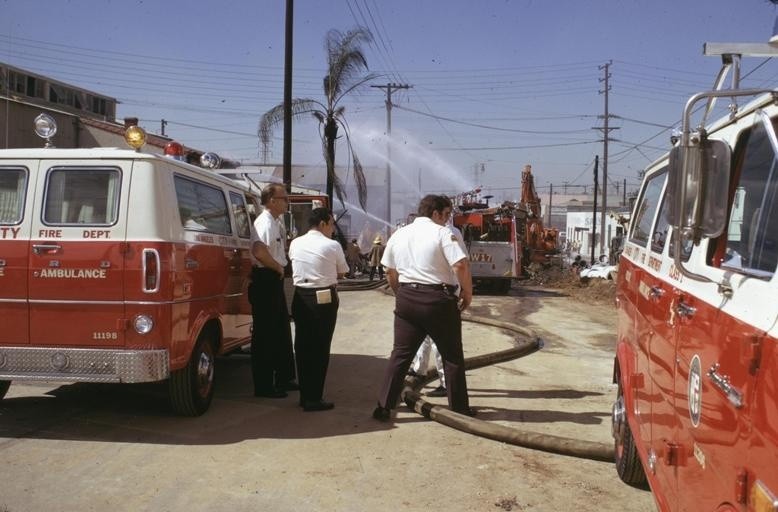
[[0, 111, 331, 417], [448, 188, 530, 296], [611, 40, 778, 512]]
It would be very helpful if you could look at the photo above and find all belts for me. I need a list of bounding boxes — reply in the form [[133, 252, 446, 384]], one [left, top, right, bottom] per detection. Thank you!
[[399, 282, 453, 290]]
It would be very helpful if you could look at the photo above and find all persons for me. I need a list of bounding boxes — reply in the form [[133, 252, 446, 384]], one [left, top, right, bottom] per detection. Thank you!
[[248, 183, 301, 401], [368, 235, 385, 282], [286, 209, 350, 411], [402, 218, 468, 398], [345, 237, 361, 279], [371, 195, 476, 422]]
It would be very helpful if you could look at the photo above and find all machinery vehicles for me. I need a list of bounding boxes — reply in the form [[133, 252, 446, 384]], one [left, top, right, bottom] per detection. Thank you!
[[511, 164, 560, 269]]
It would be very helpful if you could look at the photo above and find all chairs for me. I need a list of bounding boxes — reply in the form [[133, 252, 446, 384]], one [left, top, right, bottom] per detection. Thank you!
[[747, 209, 778, 270]]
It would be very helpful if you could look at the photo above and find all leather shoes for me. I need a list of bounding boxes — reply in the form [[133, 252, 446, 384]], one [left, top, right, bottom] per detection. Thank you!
[[299, 399, 335, 411], [406, 367, 425, 378], [255, 388, 289, 399], [456, 409, 478, 417], [372, 405, 390, 421], [424, 385, 448, 397], [276, 380, 300, 392]]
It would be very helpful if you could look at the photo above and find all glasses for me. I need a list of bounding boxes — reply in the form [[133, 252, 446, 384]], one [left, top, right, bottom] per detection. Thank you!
[[273, 196, 289, 202]]
